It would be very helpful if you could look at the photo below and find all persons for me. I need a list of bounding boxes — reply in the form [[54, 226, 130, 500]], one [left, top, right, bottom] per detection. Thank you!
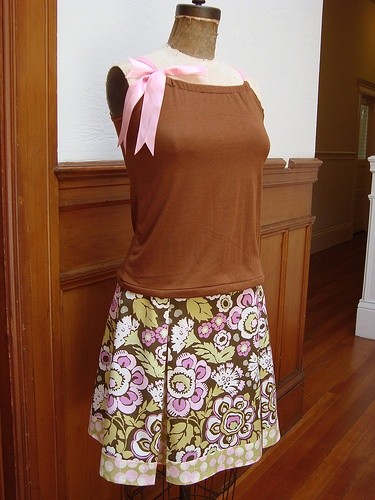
[[87, 0, 281, 486]]
